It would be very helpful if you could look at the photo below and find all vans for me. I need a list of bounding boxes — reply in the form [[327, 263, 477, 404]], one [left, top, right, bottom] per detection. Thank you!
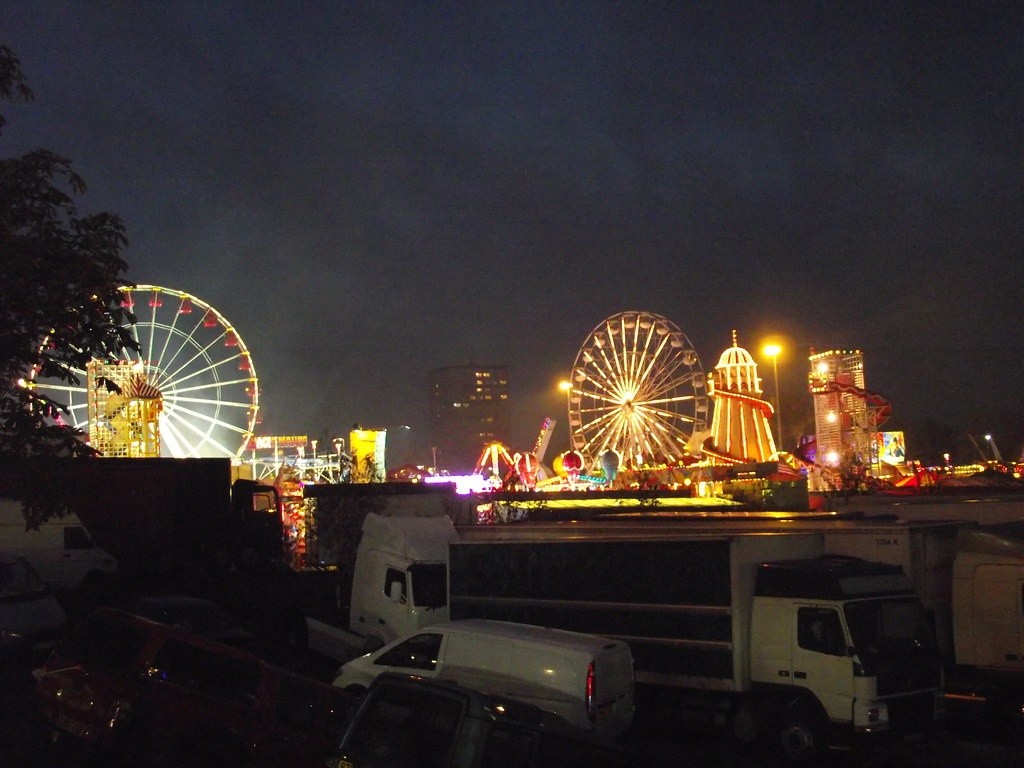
[[320, 672, 630, 767], [2, 551, 68, 678], [327, 618, 636, 745], [142, 591, 326, 673]]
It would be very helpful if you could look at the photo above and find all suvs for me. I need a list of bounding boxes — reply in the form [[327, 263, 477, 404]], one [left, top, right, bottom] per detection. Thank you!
[[28, 608, 348, 767]]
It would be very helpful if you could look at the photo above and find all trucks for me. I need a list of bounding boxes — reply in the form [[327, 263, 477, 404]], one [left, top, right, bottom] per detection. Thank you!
[[451, 515, 1024, 724], [277, 510, 462, 658], [443, 529, 958, 768], [4, 455, 287, 595], [0, 495, 121, 583]]
